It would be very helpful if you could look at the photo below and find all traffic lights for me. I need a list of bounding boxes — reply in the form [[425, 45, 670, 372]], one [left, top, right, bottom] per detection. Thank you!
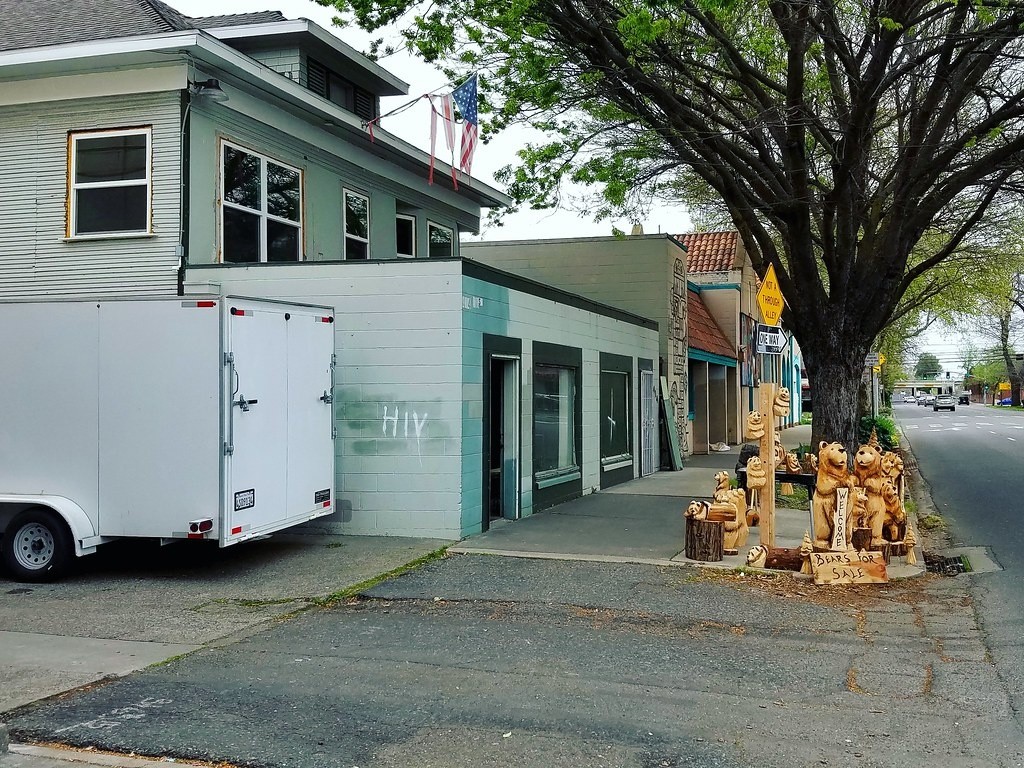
[[946, 372, 950, 379], [922, 370, 928, 380], [984, 385, 989, 390]]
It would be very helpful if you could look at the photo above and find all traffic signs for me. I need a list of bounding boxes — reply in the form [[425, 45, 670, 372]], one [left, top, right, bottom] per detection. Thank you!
[[756, 324, 789, 354]]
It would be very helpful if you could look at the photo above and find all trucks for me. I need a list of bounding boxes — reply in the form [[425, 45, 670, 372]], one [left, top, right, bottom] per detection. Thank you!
[[0, 294, 336, 583]]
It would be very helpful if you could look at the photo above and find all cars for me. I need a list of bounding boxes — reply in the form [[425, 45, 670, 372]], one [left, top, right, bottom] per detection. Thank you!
[[917, 397, 925, 406], [533, 393, 575, 443], [906, 397, 916, 403], [904, 397, 908, 402], [957, 396, 969, 405], [997, 397, 1012, 405], [932, 394, 955, 412], [923, 396, 936, 407]]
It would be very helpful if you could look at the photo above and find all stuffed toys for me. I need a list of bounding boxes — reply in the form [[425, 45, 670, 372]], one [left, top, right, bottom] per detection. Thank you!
[[682, 386, 906, 570]]
